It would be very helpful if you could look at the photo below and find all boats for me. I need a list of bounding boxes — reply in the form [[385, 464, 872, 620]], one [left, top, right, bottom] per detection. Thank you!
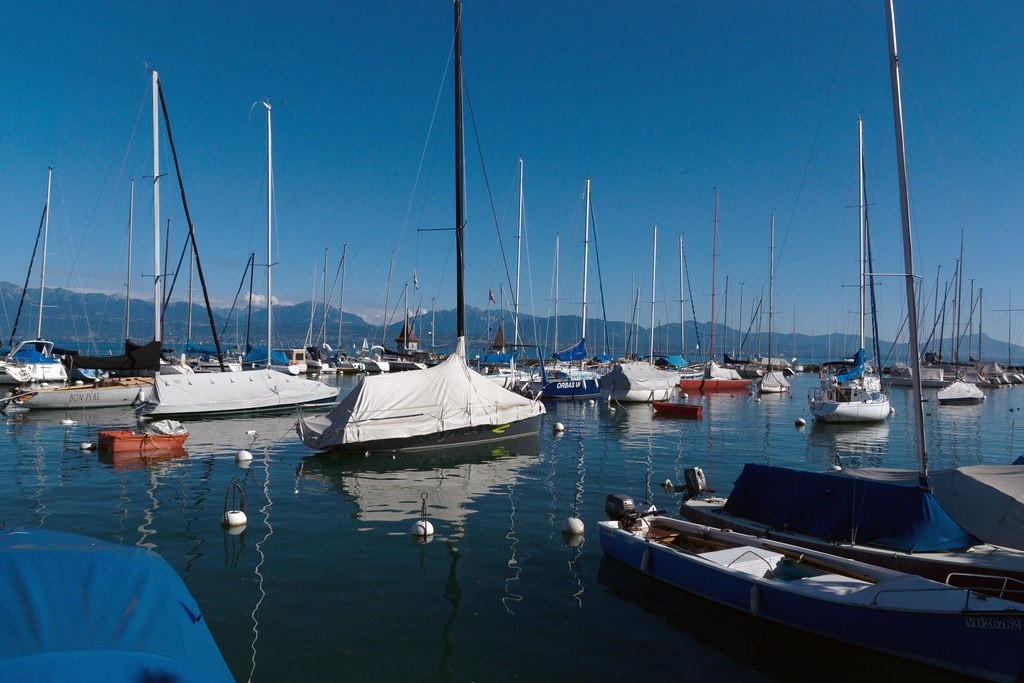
[[0, 527, 237, 683], [598, 491, 1023, 683], [98, 429, 190, 453], [650, 399, 704, 420]]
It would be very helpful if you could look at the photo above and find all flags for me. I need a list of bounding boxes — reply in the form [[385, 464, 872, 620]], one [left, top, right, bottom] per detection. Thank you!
[[490, 288, 496, 304], [413, 267, 418, 290]]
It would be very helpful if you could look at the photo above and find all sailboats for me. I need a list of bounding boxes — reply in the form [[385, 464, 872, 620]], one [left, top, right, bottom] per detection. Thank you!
[[294, 1, 550, 455], [659, 0, 1024, 601], [129, 69, 343, 422], [804, 106, 896, 425], [0, 0, 1024, 412]]
